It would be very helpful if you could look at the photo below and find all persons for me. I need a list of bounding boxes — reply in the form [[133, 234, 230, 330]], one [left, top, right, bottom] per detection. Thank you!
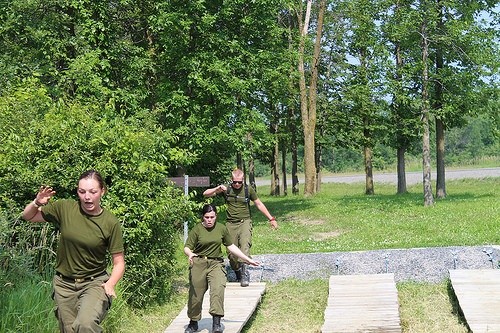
[[203, 169, 278, 288], [23, 170, 127, 333], [183, 204, 260, 333]]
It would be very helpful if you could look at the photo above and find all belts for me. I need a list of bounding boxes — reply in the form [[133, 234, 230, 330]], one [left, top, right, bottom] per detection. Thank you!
[[199, 255, 222, 260], [56, 271, 108, 284], [227, 220, 244, 223]]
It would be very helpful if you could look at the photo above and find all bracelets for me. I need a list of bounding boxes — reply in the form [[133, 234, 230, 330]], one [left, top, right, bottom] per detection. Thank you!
[[34, 199, 40, 208], [269, 218, 276, 221]]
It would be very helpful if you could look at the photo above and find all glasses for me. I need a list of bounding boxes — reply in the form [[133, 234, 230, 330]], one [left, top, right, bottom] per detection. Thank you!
[[233, 181, 242, 184]]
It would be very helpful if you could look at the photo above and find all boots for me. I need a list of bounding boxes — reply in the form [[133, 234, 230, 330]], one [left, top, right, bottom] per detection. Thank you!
[[241, 271, 249, 287], [235, 270, 241, 282], [213, 314, 220, 333], [185, 319, 198, 333]]
